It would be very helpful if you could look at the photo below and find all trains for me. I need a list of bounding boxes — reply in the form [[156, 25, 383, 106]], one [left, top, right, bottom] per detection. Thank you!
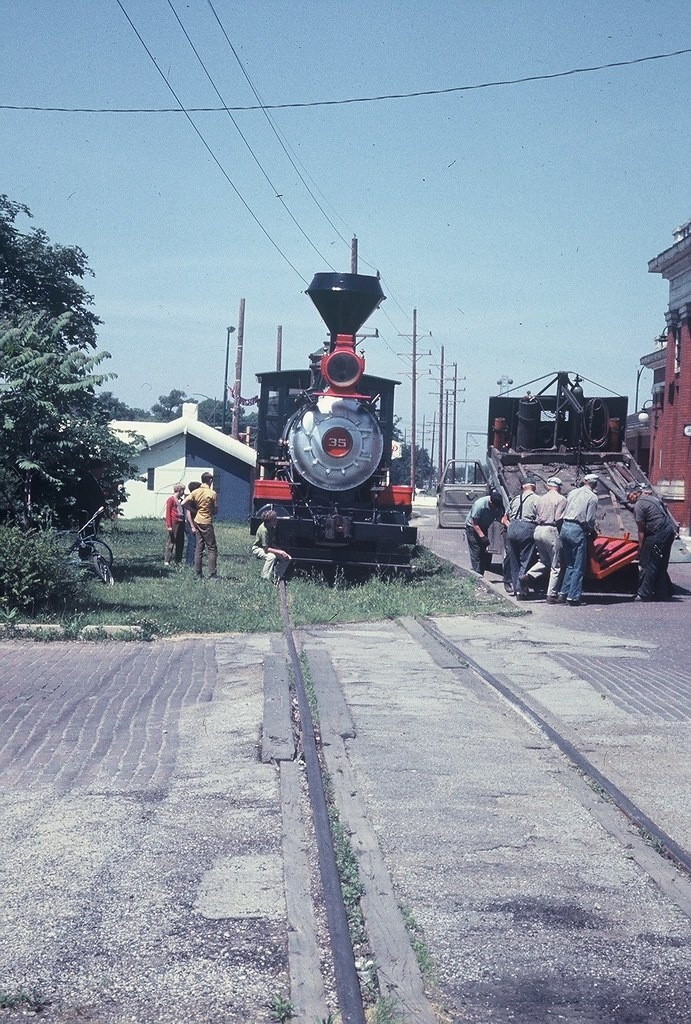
[[248, 271, 419, 582]]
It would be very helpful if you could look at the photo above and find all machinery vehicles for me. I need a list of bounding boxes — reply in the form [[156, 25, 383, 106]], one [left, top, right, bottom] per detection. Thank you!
[[436, 368, 691, 599]]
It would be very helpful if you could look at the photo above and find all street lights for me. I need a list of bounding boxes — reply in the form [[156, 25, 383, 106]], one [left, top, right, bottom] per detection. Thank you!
[[221, 325, 237, 434], [497, 375, 513, 397], [193, 392, 216, 430]]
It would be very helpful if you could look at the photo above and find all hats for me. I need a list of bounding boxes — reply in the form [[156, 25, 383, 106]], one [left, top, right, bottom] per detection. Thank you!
[[491, 492, 503, 508], [584, 474, 598, 483], [547, 477, 563, 486], [624, 481, 642, 501], [521, 477, 536, 489]]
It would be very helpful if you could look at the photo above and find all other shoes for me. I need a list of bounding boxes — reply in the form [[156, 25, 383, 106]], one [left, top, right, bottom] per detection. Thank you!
[[164, 561, 170, 566], [516, 591, 529, 600], [518, 573, 536, 589], [557, 594, 567, 602], [569, 600, 581, 606], [504, 582, 514, 595], [546, 596, 561, 604], [631, 594, 643, 601]]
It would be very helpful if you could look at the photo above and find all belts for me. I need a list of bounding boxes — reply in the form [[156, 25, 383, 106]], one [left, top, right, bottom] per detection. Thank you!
[[538, 523, 557, 527]]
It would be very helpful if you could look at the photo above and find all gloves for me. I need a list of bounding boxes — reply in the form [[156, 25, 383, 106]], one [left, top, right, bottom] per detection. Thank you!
[[479, 535, 490, 548]]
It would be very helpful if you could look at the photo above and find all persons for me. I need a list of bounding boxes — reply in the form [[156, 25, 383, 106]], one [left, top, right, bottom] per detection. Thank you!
[[502, 476, 541, 599], [465, 492, 511, 576], [625, 479, 677, 603], [179, 469, 219, 581], [253, 510, 293, 584], [183, 481, 202, 566], [165, 483, 185, 566], [554, 474, 599, 607], [518, 477, 567, 604]]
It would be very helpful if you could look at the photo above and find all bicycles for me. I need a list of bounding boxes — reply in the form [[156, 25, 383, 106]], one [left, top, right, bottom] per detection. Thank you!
[[67, 510, 115, 586]]
